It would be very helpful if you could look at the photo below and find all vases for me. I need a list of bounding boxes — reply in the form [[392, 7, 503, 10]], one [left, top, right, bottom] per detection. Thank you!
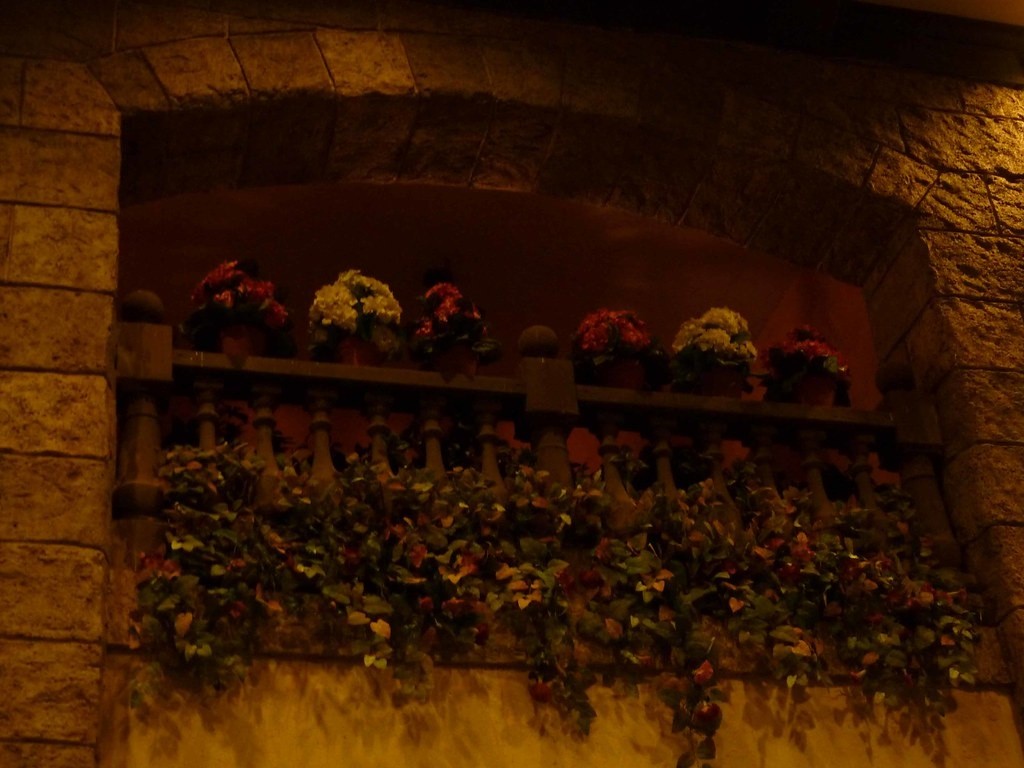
[[696, 358, 746, 398], [789, 376, 837, 407], [439, 345, 480, 377], [210, 315, 266, 357], [333, 335, 377, 366], [591, 356, 645, 392]]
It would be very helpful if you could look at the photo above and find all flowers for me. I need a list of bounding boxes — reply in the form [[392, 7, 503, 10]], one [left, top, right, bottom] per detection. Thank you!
[[757, 324, 852, 409], [674, 307, 761, 399], [571, 310, 674, 394], [407, 266, 504, 372], [178, 266, 289, 356], [306, 265, 405, 367]]
